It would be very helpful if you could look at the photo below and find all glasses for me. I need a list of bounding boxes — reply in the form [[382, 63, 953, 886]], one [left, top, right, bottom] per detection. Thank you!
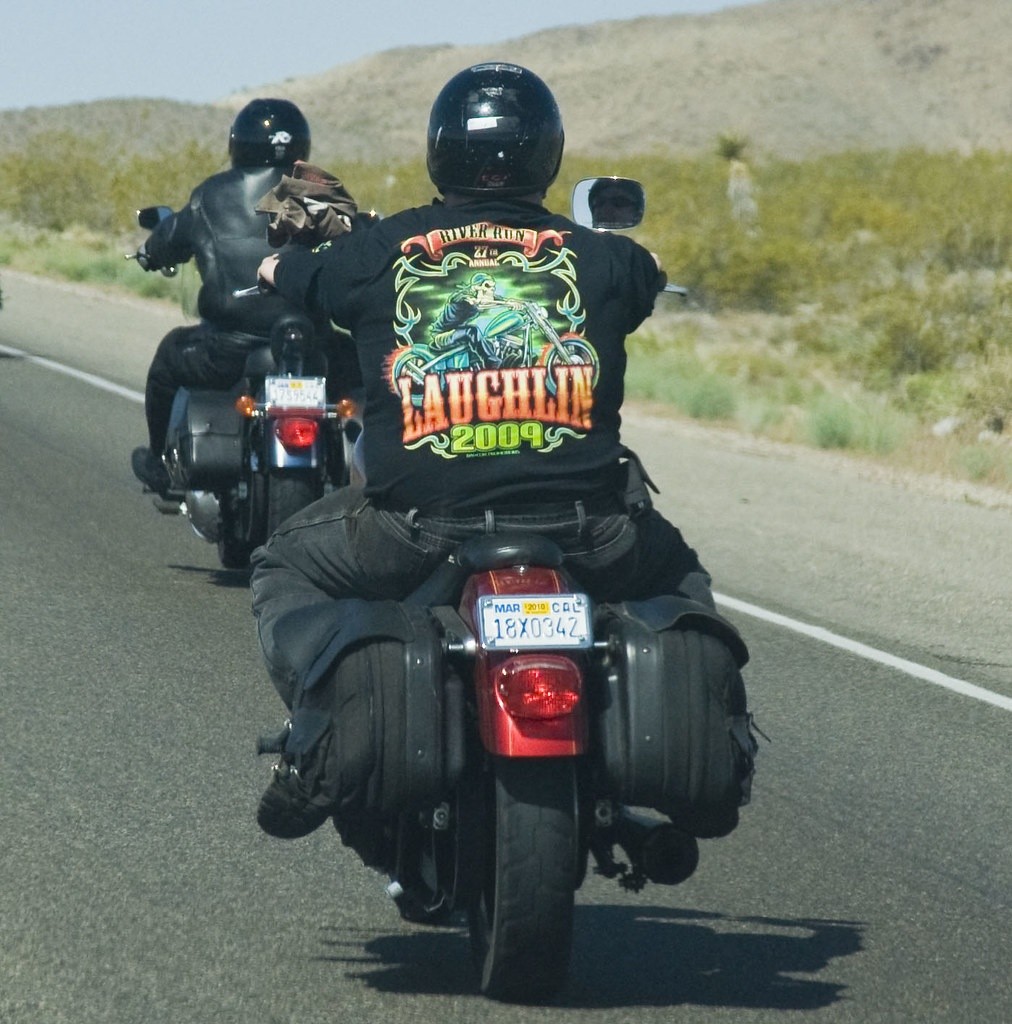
[[591, 194, 638, 210]]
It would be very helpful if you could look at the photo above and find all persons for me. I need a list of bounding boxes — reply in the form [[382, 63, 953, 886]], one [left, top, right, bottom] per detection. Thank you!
[[587, 177, 646, 230], [131, 99, 354, 491], [255, 62, 719, 837]]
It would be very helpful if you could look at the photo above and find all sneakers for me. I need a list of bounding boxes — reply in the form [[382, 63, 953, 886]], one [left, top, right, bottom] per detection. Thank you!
[[130, 445, 186, 501]]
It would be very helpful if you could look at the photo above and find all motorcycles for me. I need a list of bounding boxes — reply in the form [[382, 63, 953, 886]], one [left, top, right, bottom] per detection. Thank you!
[[233, 174, 775, 998], [124, 203, 364, 577]]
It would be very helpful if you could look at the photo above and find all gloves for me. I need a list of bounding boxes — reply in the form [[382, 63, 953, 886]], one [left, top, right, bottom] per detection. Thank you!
[[136, 243, 172, 273], [257, 253, 282, 289]]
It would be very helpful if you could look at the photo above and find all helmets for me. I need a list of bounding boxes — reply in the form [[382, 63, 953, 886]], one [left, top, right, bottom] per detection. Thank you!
[[425, 61, 565, 198], [588, 178, 645, 230], [228, 98, 312, 168]]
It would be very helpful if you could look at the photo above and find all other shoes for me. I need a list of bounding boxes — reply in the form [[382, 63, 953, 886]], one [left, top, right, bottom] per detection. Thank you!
[[255, 663, 347, 840]]
